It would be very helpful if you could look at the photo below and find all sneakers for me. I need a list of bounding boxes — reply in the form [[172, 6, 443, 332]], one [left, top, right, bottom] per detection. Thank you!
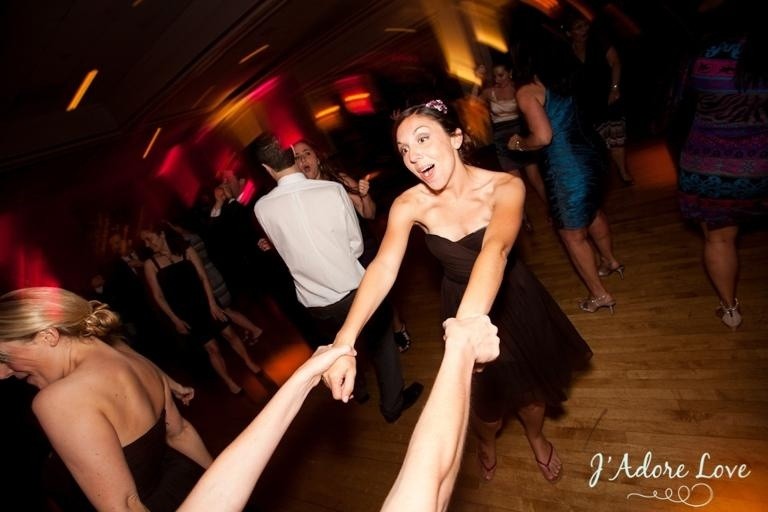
[[522, 215, 535, 234], [247, 327, 265, 347], [240, 329, 249, 342]]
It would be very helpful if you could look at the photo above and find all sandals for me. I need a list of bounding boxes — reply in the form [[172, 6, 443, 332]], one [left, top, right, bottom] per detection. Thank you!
[[226, 382, 248, 396], [395, 321, 412, 354], [245, 363, 264, 377]]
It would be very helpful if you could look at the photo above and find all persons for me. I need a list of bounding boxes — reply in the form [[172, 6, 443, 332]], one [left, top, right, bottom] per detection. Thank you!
[[1, 1, 768, 510]]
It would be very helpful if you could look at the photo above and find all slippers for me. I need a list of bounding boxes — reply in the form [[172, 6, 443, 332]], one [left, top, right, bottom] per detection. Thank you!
[[531, 439, 564, 485], [474, 440, 499, 483]]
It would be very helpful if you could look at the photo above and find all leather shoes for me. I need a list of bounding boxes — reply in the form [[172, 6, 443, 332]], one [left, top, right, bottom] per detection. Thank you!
[[379, 379, 425, 424], [356, 392, 371, 404], [616, 168, 637, 186]]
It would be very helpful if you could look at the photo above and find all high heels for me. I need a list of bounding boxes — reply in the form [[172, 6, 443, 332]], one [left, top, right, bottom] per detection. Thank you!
[[596, 262, 626, 282], [577, 292, 616, 316], [714, 296, 745, 336]]
